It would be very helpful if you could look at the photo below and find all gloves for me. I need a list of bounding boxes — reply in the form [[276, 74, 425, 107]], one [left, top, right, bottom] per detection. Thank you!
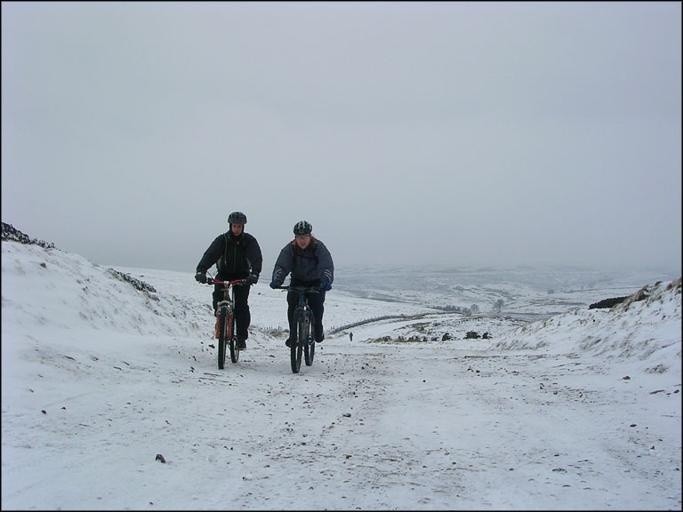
[[321, 281, 331, 289], [249, 274, 258, 283], [195, 271, 206, 284], [271, 280, 282, 289]]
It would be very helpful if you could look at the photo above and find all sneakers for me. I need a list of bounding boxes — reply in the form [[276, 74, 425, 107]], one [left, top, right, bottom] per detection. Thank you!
[[315, 326, 324, 343], [285, 337, 296, 347], [236, 336, 246, 348]]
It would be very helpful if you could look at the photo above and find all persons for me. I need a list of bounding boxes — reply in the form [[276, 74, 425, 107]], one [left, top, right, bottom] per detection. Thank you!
[[270, 221, 334, 348], [195, 212, 262, 347]]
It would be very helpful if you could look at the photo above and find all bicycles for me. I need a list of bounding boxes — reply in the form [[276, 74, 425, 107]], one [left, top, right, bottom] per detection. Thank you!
[[271, 284, 315, 373], [202, 276, 252, 371]]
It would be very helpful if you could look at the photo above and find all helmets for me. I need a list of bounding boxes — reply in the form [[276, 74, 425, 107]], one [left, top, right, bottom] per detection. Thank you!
[[227, 211, 247, 223], [293, 221, 312, 234]]
